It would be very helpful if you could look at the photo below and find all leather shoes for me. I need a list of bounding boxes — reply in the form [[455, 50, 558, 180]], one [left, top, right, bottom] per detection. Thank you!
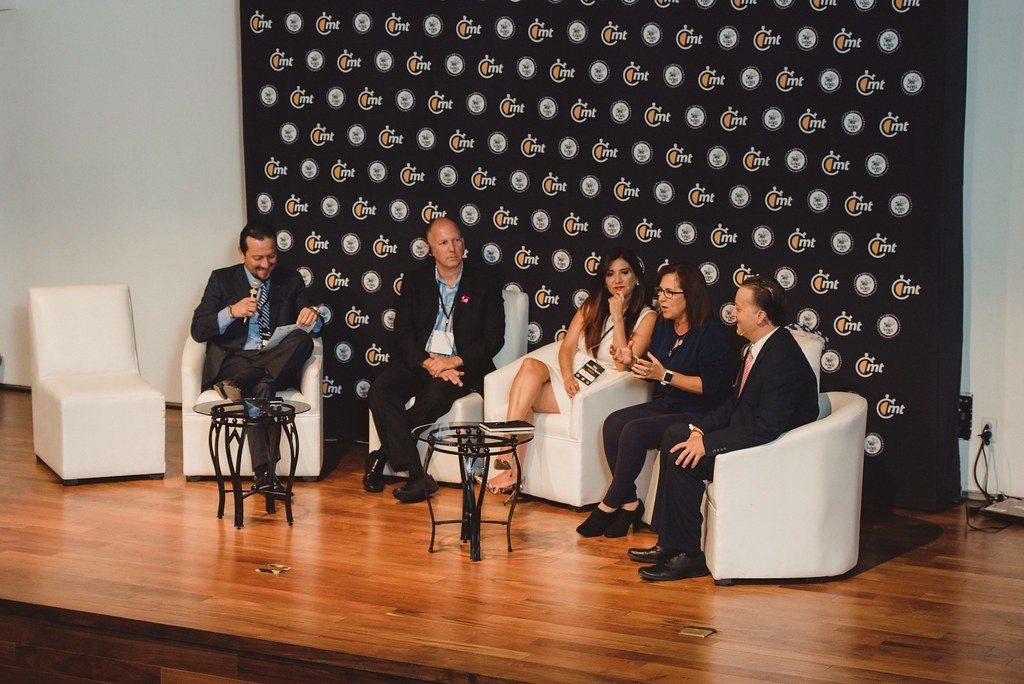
[[213, 379, 245, 401], [393, 475, 439, 501], [638, 553, 709, 581], [251, 470, 295, 496], [628, 543, 682, 563], [363, 450, 385, 492]]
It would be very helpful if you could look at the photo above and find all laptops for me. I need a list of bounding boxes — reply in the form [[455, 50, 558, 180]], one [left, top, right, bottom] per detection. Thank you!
[[979, 498, 1024, 524]]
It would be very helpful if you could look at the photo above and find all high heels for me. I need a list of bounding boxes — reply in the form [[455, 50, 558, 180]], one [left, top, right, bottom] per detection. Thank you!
[[474, 475, 526, 505], [576, 506, 620, 536], [494, 451, 517, 477], [604, 498, 645, 537]]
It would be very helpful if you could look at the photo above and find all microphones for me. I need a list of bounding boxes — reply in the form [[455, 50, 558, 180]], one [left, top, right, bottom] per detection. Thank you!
[[242, 281, 260, 326]]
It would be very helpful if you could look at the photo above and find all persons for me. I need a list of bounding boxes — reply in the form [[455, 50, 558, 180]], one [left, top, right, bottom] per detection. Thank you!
[[363, 216, 505, 501], [191, 220, 326, 499], [628, 275, 821, 581], [577, 262, 728, 539], [474, 247, 658, 505]]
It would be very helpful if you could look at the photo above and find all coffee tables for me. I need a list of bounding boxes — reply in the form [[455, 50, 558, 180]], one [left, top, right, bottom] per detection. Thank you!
[[411, 422, 534, 559], [194, 398, 311, 529]]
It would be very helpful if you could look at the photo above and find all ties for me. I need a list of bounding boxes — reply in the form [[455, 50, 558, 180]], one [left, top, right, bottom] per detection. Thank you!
[[255, 284, 271, 348], [738, 352, 753, 398]]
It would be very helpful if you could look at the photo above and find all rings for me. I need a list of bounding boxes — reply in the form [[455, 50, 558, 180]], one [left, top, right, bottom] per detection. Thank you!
[[644, 373, 646, 376]]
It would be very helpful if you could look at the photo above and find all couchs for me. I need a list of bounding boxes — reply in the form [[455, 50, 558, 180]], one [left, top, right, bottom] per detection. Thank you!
[[368, 288, 868, 586], [182, 335, 324, 482]]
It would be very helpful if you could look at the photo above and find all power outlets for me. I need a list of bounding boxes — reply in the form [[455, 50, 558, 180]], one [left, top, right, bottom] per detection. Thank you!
[[981, 417, 997, 441]]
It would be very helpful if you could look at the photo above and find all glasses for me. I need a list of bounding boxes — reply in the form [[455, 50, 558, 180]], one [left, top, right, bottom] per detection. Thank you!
[[654, 287, 684, 299]]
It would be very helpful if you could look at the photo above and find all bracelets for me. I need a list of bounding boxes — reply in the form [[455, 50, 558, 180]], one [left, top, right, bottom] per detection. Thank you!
[[229, 306, 234, 317], [690, 429, 704, 436]]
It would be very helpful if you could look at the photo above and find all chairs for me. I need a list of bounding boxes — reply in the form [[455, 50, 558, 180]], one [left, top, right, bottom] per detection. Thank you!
[[27, 285, 169, 486]]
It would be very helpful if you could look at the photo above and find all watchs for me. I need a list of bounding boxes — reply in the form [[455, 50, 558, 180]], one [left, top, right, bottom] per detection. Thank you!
[[660, 369, 673, 386]]
